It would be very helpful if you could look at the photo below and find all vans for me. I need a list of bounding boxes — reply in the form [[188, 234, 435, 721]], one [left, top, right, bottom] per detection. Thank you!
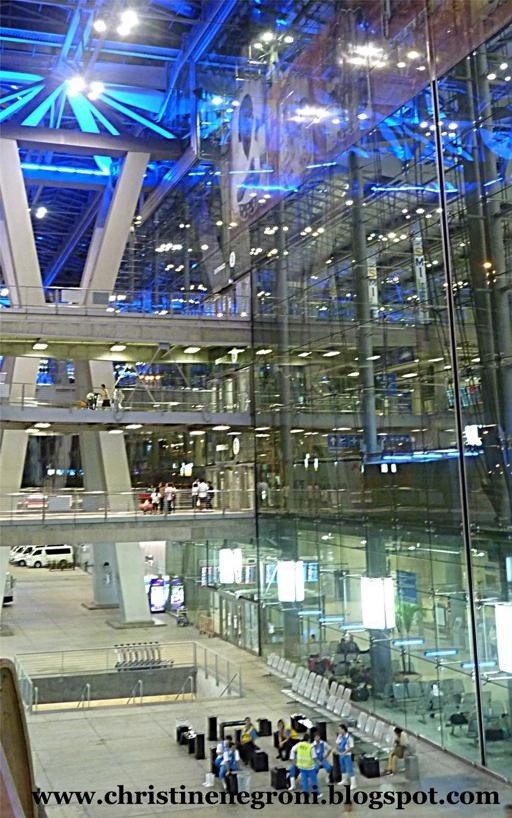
[[7, 544, 75, 567]]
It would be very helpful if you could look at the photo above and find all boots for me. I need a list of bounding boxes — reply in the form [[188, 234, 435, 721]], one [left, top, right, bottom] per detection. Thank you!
[[202, 773, 216, 788], [325, 773, 357, 790], [315, 793, 324, 799], [288, 777, 297, 791]]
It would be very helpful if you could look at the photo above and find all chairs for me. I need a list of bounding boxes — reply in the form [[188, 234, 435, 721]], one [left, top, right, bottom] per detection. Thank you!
[[256, 652, 419, 774]]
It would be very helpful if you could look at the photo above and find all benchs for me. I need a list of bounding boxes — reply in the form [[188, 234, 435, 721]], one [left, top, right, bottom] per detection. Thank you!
[[272, 762, 334, 790], [207, 729, 269, 796]]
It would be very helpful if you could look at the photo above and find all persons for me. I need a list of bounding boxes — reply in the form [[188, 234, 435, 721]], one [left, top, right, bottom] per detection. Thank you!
[[86, 392, 99, 410], [346, 635, 361, 664], [307, 634, 320, 657], [97, 382, 111, 410], [137, 477, 215, 514], [230, 90, 325, 220], [335, 638, 348, 661], [211, 715, 410, 799]]
